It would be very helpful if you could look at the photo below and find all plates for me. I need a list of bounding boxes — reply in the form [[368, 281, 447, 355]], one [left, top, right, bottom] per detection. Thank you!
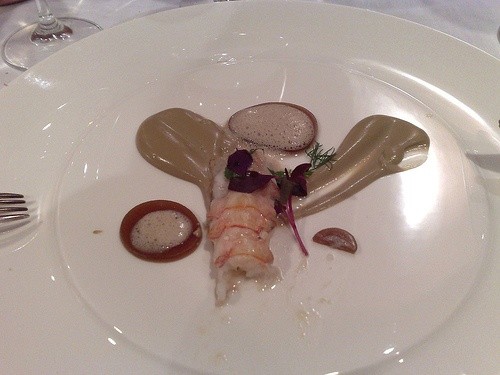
[[0, 0, 499, 375]]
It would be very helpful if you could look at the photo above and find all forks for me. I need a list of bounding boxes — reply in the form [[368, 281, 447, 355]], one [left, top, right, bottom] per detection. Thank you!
[[0, 193, 30, 223]]
